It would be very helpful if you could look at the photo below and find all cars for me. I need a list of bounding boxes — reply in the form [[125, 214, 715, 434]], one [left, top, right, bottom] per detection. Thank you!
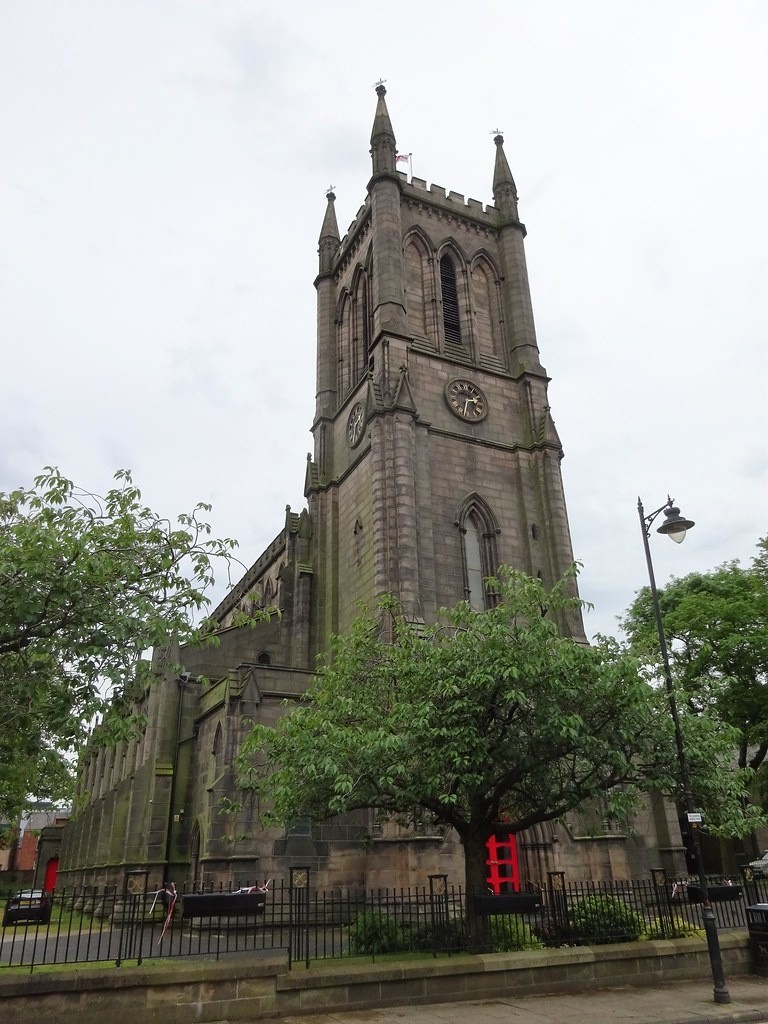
[[1, 890, 51, 927]]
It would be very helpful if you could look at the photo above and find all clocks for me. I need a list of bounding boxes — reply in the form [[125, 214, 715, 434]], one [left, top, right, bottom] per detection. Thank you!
[[445, 378, 488, 423], [346, 401, 366, 448]]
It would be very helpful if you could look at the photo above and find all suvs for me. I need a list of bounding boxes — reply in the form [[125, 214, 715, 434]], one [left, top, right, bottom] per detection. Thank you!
[[750, 850, 768, 878]]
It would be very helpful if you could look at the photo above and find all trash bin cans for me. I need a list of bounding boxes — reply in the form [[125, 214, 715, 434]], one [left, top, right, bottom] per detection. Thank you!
[[745, 903, 767, 978]]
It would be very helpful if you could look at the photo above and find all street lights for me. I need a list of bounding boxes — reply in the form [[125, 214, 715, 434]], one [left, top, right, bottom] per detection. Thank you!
[[635, 493, 735, 1007]]
